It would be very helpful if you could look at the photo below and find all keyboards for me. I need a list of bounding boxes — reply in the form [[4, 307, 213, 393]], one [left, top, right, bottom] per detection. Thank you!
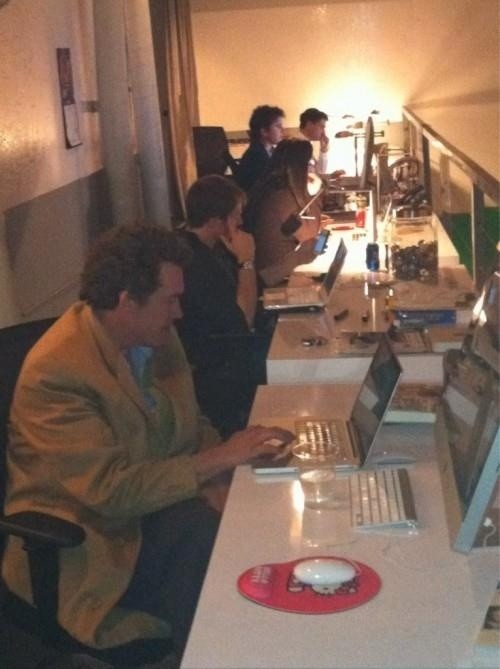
[[350, 468, 418, 529]]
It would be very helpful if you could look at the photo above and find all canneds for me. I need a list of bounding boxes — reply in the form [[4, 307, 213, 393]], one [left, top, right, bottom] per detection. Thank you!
[[355, 209, 365, 227], [365, 242, 380, 272]]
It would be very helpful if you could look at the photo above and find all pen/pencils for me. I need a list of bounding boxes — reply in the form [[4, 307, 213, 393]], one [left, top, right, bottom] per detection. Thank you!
[[334, 309, 349, 320], [332, 227, 353, 230]]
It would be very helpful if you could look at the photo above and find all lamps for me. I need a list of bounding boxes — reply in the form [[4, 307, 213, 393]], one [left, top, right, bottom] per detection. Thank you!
[[334, 129, 385, 177]]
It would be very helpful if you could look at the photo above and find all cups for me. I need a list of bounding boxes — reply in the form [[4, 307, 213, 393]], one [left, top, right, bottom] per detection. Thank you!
[[291, 440, 339, 507], [298, 503, 344, 560]]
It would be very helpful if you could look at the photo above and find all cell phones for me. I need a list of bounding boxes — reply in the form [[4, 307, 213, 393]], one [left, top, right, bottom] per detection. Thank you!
[[314, 229, 330, 254]]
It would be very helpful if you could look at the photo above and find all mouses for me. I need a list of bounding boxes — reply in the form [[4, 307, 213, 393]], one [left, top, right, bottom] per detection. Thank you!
[[293, 558, 357, 586]]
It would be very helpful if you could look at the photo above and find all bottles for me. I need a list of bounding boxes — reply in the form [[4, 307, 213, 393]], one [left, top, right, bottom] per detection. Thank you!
[[356, 193, 367, 227]]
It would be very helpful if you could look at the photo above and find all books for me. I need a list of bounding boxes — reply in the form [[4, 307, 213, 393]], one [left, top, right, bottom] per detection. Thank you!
[[380, 382, 445, 426]]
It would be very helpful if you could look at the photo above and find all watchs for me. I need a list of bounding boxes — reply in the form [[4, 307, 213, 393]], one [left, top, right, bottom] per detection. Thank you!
[[238, 258, 259, 271]]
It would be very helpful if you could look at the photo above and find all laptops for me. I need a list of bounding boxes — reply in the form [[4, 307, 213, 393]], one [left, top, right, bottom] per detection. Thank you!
[[263, 238, 348, 310], [251, 332, 404, 475]]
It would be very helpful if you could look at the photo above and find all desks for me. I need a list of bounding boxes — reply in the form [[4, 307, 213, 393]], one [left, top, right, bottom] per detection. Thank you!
[[291, 211, 461, 275], [261, 260, 480, 383], [175, 377, 500, 669]]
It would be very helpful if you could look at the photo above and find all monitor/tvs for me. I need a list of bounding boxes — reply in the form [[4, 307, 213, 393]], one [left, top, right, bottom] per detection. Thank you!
[[360, 116, 374, 189], [369, 190, 377, 244], [434, 336, 500, 555]]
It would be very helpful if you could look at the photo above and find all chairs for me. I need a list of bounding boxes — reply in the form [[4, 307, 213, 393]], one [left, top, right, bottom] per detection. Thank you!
[[190, 124, 241, 181], [1, 312, 183, 668]]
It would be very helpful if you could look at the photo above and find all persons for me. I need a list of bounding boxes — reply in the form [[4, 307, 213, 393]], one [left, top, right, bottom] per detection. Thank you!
[[288, 108, 346, 179], [3, 217, 296, 669], [231, 104, 286, 189], [238, 137, 334, 259], [172, 174, 329, 370]]
